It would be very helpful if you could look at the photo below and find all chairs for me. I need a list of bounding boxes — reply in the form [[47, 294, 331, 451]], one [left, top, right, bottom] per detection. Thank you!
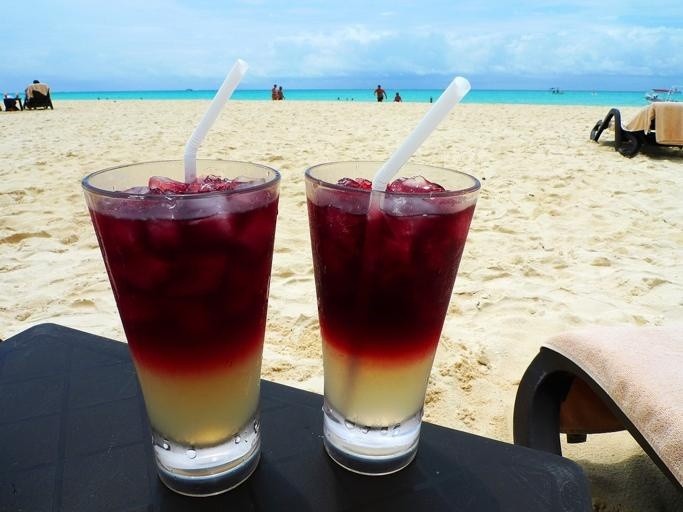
[[23, 84, 52, 109], [590, 102, 683, 158]]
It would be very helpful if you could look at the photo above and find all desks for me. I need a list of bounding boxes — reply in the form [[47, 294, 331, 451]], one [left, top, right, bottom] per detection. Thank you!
[[0, 323, 591, 511]]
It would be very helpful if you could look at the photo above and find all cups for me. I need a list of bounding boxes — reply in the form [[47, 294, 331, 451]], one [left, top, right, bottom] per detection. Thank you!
[[73, 156, 283, 497], [301, 156, 482, 477]]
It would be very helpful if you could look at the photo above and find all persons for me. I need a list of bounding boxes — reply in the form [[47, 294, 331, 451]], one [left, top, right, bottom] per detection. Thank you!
[[338, 96, 354, 101], [25, 79, 40, 92], [271, 84, 279, 100], [393, 92, 402, 102], [430, 95, 432, 103], [550, 87, 559, 95], [278, 86, 284, 100], [373, 85, 387, 102]]
